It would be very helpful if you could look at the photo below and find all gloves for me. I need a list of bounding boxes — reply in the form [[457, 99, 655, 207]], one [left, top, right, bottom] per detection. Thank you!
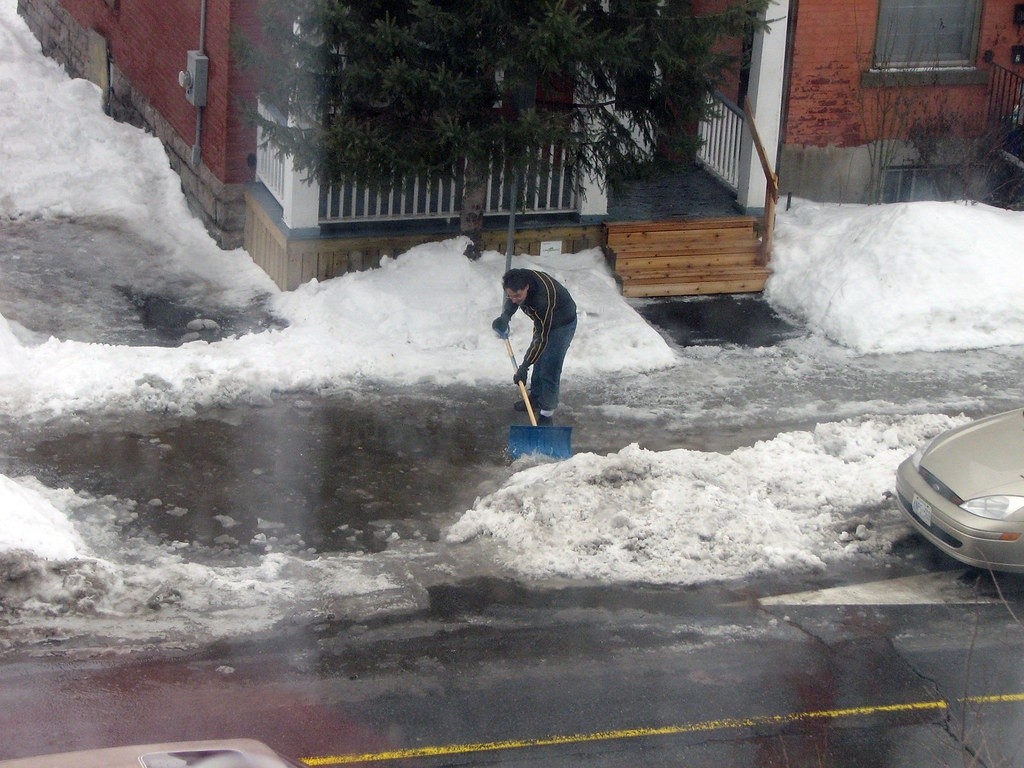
[[513, 365, 529, 386], [492, 312, 511, 333]]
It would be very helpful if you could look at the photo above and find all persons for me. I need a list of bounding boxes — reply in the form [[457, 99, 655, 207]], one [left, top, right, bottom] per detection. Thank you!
[[492, 267, 577, 427]]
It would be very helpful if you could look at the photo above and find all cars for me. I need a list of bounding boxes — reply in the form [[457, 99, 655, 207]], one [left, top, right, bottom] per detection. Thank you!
[[893, 408, 1023, 574]]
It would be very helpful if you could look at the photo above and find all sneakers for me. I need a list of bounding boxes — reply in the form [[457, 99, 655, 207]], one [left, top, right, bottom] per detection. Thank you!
[[514, 393, 540, 411], [537, 415, 553, 426]]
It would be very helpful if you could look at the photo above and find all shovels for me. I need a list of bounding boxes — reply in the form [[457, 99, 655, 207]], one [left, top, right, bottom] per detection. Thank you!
[[494, 326, 571, 465]]
